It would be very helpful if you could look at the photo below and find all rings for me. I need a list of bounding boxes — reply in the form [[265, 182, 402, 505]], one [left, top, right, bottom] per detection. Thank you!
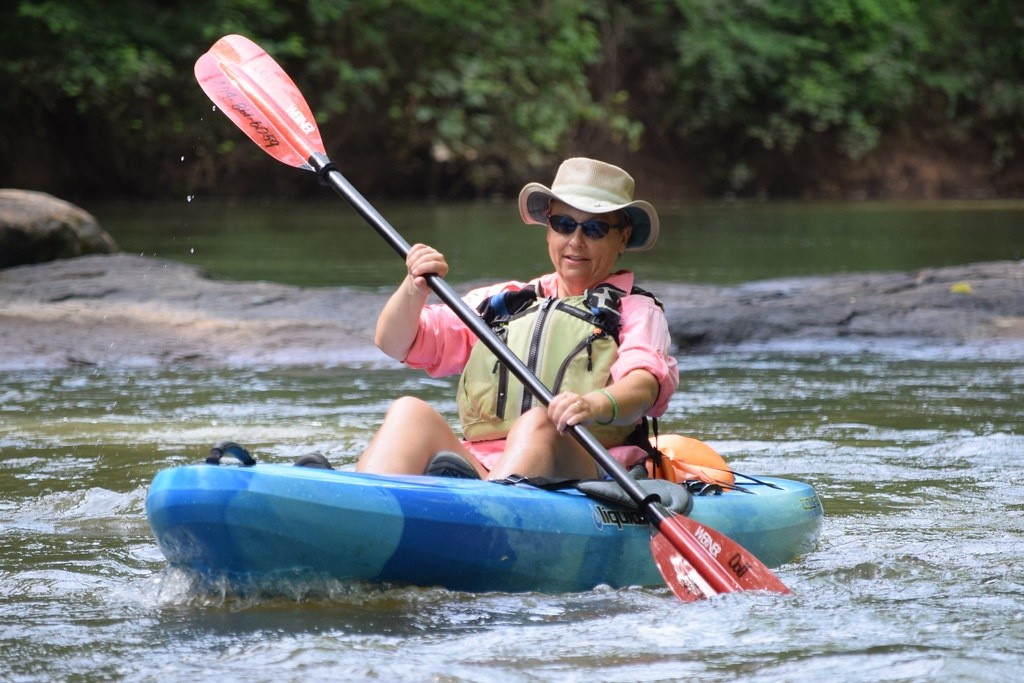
[[576, 399, 583, 409]]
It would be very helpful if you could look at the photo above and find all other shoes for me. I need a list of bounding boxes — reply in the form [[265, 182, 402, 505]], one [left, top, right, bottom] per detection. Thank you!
[[428, 450, 483, 480], [294, 451, 332, 470]]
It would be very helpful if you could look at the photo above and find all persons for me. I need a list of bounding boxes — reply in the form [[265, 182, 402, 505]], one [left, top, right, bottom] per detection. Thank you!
[[352, 156, 679, 475]]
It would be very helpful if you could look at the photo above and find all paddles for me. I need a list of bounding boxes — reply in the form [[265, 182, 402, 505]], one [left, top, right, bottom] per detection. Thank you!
[[191, 33, 791, 608]]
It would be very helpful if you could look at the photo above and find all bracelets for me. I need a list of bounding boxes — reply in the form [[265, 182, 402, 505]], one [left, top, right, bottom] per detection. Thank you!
[[592, 388, 618, 425]]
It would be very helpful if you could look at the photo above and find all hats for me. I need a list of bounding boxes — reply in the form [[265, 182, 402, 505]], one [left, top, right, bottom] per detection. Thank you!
[[518, 156, 660, 251]]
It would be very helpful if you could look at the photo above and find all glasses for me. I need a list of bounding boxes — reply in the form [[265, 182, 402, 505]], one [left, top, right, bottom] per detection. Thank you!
[[544, 212, 625, 240]]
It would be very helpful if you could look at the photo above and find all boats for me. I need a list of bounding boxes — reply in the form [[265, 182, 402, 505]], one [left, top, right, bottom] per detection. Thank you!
[[142, 440, 827, 606]]
